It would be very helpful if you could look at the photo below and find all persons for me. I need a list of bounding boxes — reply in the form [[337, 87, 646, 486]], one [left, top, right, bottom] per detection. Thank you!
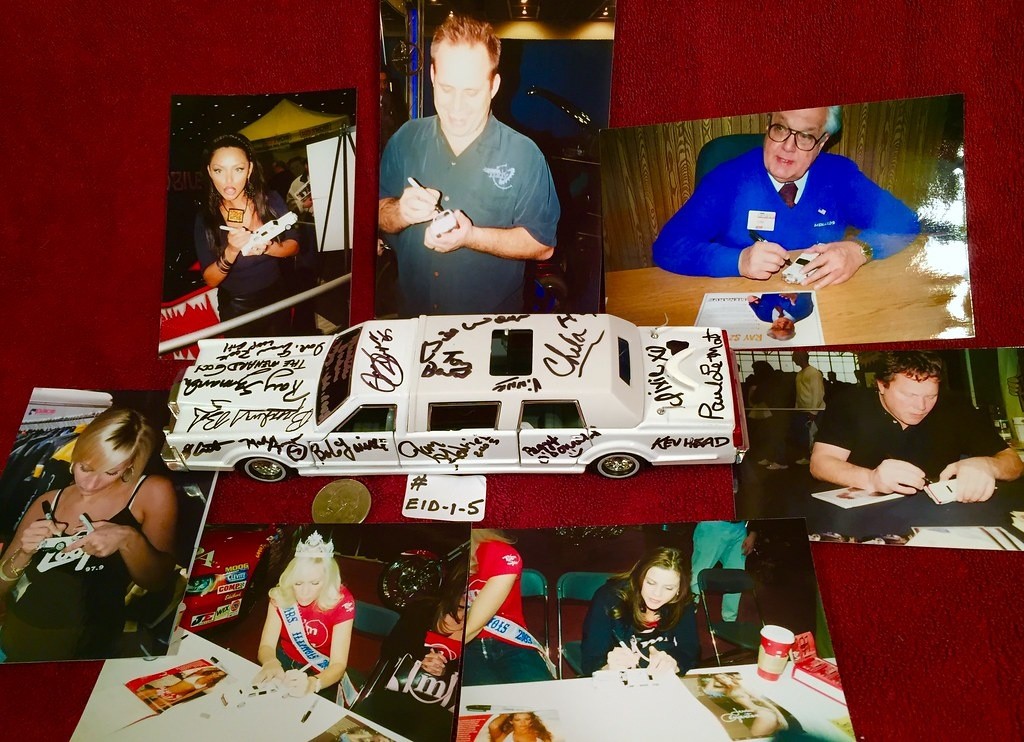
[[745, 294, 815, 341], [735, 349, 1024, 504], [691, 521, 749, 623], [463, 526, 558, 683], [377, 237, 385, 257], [259, 532, 464, 742], [191, 131, 304, 337], [138, 666, 226, 711], [742, 521, 818, 640], [650, 104, 922, 289], [578, 545, 700, 679], [379, 69, 399, 156], [264, 158, 314, 225], [378, 16, 561, 315], [487, 712, 553, 742], [704, 672, 780, 736], [0, 404, 177, 661]]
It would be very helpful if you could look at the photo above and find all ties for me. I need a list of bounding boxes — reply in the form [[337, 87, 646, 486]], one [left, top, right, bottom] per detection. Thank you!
[[778, 181, 798, 207], [775, 306, 785, 318]]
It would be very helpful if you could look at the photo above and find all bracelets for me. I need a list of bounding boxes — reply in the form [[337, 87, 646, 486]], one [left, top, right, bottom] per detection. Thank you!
[[313, 674, 320, 694], [215, 251, 233, 274], [0, 548, 32, 581]]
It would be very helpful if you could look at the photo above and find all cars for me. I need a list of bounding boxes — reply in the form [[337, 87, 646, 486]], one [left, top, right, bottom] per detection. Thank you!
[[157, 314, 749, 484], [177, 523, 299, 632]]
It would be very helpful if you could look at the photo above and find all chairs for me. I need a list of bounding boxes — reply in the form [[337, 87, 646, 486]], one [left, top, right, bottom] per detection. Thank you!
[[555, 571, 619, 680], [693, 133, 765, 192], [521, 568, 550, 658], [349, 598, 400, 709], [697, 567, 766, 667], [362, 603, 436, 698]]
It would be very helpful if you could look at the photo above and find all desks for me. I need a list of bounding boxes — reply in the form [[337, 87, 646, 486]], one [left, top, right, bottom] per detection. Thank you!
[[605, 228, 975, 346], [456, 657, 854, 742], [70, 627, 413, 742], [733, 463, 1024, 552]]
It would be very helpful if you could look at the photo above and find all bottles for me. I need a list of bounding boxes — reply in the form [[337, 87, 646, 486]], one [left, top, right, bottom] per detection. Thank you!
[[993, 404, 1013, 443]]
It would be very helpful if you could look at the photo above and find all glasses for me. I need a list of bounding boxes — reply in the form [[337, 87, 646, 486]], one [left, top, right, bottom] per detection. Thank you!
[[768, 116, 826, 152]]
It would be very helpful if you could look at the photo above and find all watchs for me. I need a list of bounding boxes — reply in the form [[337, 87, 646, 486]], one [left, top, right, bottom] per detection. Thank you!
[[851, 239, 873, 265]]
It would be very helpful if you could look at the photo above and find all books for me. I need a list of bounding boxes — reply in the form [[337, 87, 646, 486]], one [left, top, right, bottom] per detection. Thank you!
[[793, 658, 848, 707]]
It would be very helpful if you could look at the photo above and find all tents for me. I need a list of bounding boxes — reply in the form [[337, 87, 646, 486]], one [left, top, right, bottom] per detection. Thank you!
[[239, 98, 354, 157]]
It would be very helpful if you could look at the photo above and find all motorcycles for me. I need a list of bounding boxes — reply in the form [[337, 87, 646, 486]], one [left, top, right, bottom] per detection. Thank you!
[[376, 539, 475, 611]]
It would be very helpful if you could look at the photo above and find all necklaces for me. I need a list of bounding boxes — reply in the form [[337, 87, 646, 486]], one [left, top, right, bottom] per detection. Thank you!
[[221, 197, 248, 224]]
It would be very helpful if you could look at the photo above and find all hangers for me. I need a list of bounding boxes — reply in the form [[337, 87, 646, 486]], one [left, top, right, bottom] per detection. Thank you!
[[11, 411, 101, 452]]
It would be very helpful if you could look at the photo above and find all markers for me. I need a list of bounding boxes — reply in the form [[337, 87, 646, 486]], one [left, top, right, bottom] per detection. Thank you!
[[220, 226, 249, 236], [617, 638, 641, 668], [751, 229, 794, 265], [80, 514, 95, 532], [299, 659, 317, 673], [407, 176, 444, 212], [41, 499, 61, 533]]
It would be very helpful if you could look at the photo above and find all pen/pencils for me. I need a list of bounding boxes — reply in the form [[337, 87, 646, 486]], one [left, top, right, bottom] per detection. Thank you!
[[890, 455, 933, 484], [302, 699, 320, 723]]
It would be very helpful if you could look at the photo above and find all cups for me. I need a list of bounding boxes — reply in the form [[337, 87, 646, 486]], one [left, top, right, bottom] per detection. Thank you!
[[757, 625, 795, 681]]
